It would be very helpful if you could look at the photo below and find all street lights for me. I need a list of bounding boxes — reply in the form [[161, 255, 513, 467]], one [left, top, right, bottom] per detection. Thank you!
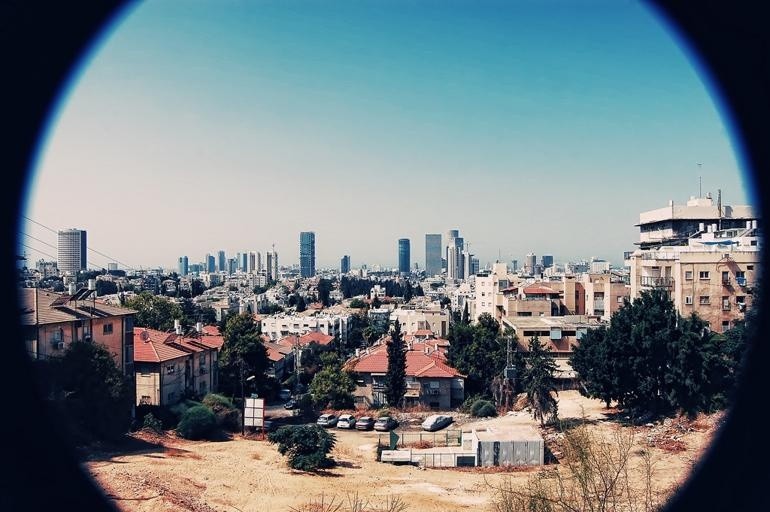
[[239, 373, 257, 438]]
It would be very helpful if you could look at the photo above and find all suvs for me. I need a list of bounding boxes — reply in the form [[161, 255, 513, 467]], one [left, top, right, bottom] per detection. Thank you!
[[336, 415, 356, 430], [317, 414, 338, 428]]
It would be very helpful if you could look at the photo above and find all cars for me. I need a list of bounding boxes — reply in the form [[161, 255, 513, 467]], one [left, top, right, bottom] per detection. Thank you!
[[355, 417, 377, 431], [275, 376, 309, 411], [421, 415, 453, 431], [374, 417, 398, 433], [331, 339, 371, 369]]
